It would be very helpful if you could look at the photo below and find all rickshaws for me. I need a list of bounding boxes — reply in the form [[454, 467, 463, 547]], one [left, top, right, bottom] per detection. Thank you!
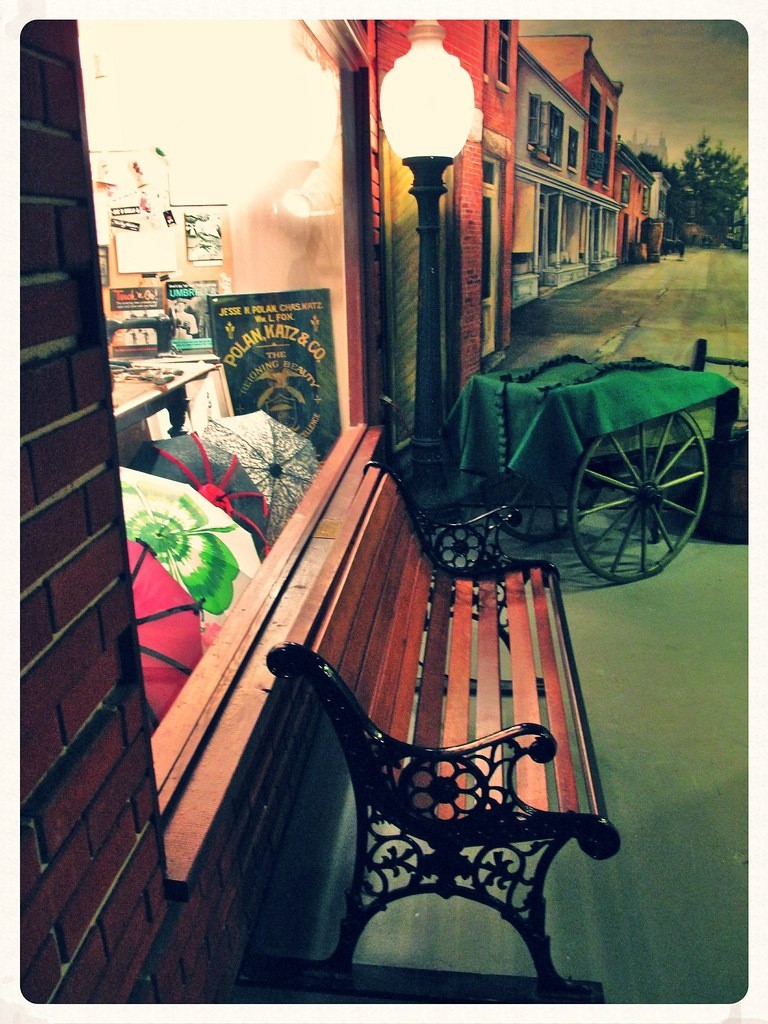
[[468, 338, 747, 584]]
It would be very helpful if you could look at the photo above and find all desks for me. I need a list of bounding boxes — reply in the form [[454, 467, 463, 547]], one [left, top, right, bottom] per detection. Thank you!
[[106, 353, 222, 438]]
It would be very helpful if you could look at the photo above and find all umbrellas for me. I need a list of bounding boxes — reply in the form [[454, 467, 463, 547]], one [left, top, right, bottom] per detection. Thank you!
[[118, 409, 319, 738]]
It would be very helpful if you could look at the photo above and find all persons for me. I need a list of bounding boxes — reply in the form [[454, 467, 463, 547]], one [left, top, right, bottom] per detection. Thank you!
[[174, 296, 201, 339]]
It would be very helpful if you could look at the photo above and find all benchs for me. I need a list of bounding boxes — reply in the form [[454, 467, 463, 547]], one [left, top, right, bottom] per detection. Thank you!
[[260, 460, 622, 1004]]
